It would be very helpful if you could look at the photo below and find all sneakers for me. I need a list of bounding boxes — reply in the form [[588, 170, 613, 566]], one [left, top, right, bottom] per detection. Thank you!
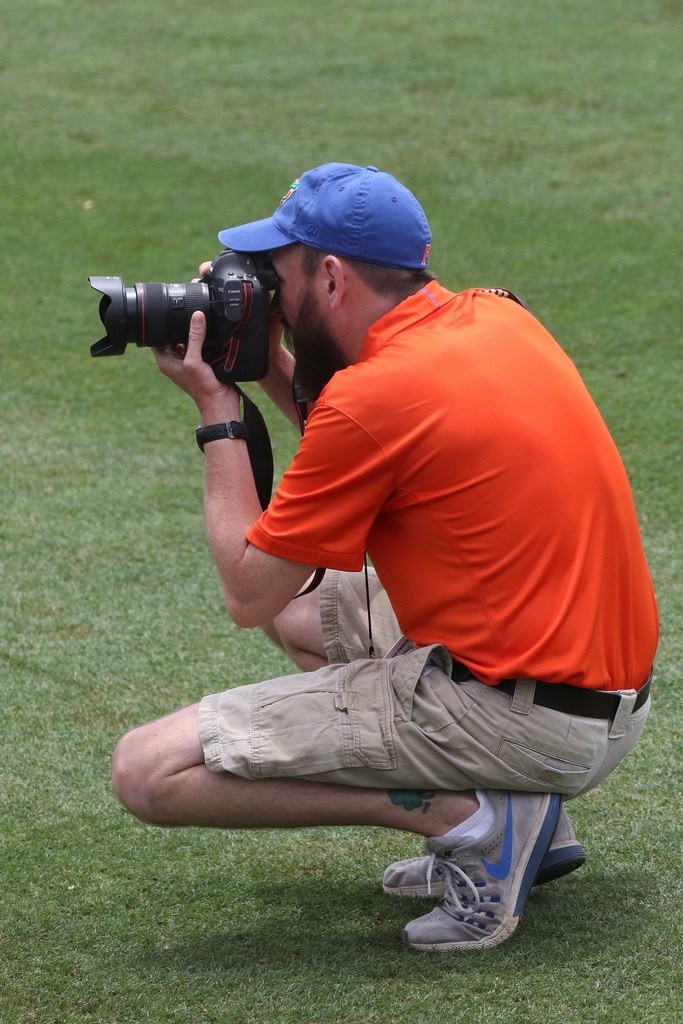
[[380, 801, 586, 898], [398, 790, 560, 951]]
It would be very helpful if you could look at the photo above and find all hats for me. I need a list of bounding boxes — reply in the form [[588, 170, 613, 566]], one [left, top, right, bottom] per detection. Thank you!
[[218, 163, 431, 269]]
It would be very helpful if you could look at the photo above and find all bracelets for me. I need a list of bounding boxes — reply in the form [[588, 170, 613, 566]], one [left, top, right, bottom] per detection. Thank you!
[[196, 420, 247, 453]]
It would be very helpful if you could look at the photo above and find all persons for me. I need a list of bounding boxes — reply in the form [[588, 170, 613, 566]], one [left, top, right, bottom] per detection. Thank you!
[[112, 160, 659, 952]]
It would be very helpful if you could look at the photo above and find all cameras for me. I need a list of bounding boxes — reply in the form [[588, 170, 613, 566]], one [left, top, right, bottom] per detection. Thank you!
[[88, 247, 280, 381]]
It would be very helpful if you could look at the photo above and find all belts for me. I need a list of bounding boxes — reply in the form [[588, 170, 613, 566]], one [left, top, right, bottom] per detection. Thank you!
[[450, 660, 653, 718]]
